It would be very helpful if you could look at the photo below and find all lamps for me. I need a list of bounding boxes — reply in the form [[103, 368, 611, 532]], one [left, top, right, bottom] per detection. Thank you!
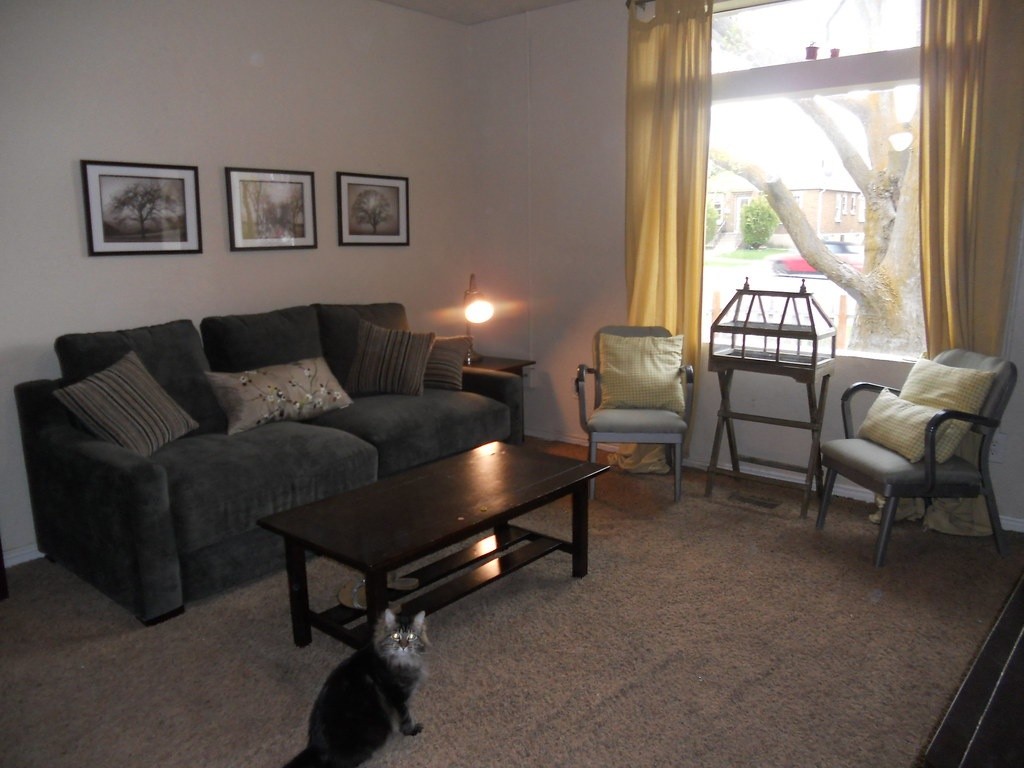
[[465, 273, 496, 361]]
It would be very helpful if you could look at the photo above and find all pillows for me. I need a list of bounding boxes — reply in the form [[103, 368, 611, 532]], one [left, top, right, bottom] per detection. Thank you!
[[52, 350, 199, 460], [857, 384, 951, 460], [424, 334, 475, 390], [203, 355, 354, 435], [596, 334, 687, 412], [896, 352, 996, 463], [346, 317, 435, 395]]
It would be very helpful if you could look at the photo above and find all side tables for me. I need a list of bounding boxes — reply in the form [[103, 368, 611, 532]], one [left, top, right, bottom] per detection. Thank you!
[[465, 355, 538, 374]]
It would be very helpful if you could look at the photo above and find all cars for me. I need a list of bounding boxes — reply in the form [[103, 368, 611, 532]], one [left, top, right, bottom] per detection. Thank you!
[[774, 240, 864, 277]]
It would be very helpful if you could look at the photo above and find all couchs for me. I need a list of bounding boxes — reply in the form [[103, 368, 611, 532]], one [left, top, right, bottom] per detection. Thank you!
[[15, 296, 522, 629]]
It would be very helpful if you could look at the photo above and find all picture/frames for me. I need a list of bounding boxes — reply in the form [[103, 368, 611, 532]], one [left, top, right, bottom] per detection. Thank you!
[[80, 160, 204, 254], [223, 165, 318, 250], [335, 171, 411, 245]]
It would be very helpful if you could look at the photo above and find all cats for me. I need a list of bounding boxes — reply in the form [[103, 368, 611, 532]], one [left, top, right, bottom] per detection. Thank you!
[[283, 608, 427, 768]]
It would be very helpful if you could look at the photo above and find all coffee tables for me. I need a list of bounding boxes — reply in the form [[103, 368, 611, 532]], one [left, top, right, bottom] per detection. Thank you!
[[257, 440, 609, 650]]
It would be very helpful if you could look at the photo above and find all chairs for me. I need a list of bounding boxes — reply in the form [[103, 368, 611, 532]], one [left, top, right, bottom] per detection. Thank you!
[[815, 345, 1017, 564], [575, 325, 694, 503]]
